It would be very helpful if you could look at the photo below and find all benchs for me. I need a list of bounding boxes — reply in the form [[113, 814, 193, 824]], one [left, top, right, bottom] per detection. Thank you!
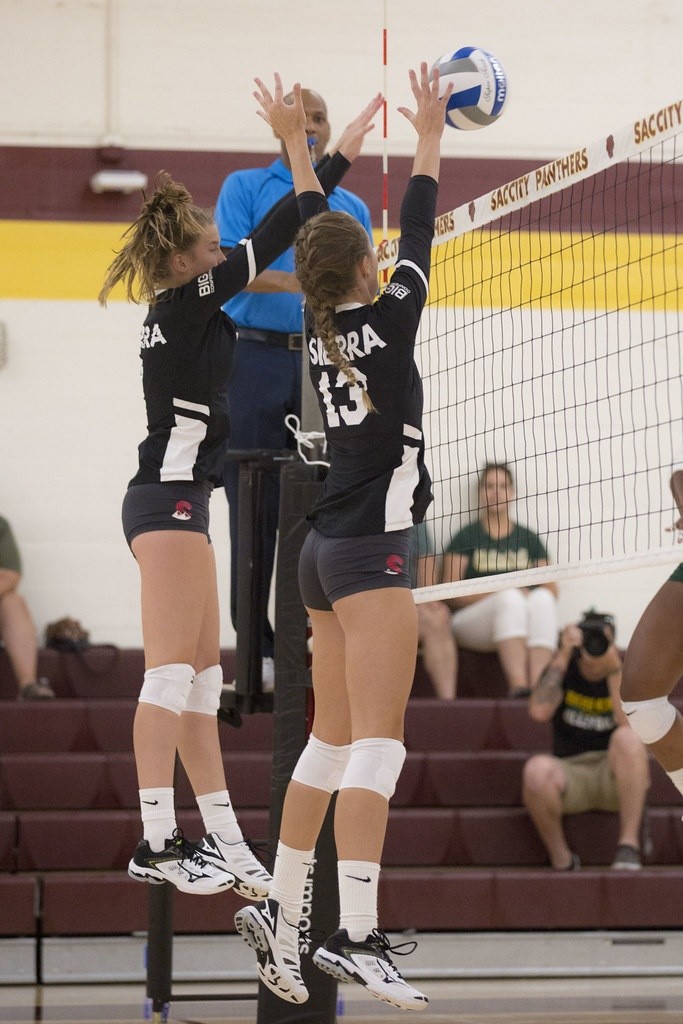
[[0, 647, 683, 931]]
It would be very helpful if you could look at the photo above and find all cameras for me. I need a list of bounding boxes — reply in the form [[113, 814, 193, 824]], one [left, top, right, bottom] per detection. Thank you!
[[575, 621, 608, 659]]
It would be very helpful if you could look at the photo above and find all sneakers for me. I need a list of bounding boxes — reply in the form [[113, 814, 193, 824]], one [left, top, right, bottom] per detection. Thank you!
[[234, 898, 326, 1004], [194, 830, 274, 901], [127, 827, 236, 895], [313, 928, 428, 1012]]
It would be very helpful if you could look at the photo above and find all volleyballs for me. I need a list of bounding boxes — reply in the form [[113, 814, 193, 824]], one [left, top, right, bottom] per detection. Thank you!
[[427, 44, 511, 132]]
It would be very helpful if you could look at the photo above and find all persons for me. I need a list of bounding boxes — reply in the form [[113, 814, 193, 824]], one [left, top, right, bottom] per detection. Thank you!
[[618, 470, 683, 796], [408, 518, 459, 699], [213, 87, 377, 688], [522, 609, 649, 874], [98, 91, 383, 902], [442, 464, 559, 698], [233, 62, 453, 1010], [0, 517, 55, 701]]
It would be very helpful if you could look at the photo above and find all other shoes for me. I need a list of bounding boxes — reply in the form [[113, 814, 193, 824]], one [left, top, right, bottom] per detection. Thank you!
[[16, 682, 52, 701], [610, 844, 642, 871], [552, 853, 581, 871], [263, 657, 278, 694]]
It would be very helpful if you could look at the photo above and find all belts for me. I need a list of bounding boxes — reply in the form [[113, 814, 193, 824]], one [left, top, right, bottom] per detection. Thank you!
[[236, 325, 303, 351]]
